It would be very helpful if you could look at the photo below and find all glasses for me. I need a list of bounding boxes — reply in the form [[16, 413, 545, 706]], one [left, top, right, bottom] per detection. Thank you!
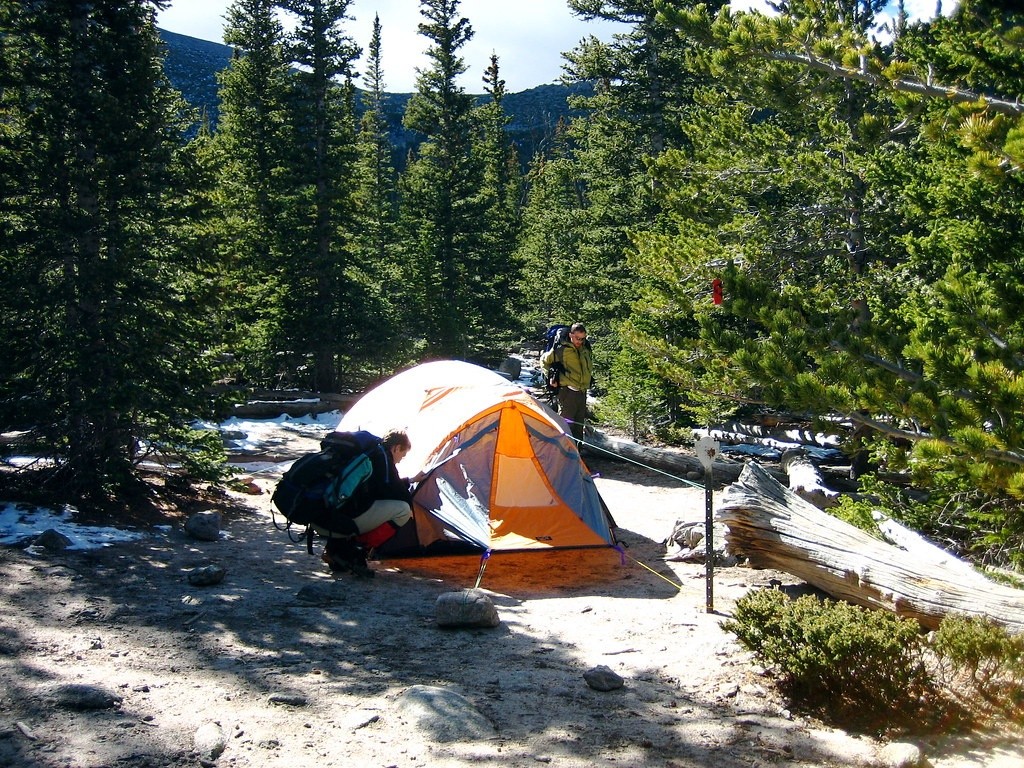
[[572, 333, 585, 341]]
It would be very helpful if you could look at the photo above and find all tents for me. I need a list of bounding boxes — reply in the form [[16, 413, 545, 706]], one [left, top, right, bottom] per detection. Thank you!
[[337, 360, 623, 553]]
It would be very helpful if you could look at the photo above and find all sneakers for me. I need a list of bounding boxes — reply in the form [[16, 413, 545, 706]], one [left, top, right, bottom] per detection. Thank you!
[[321, 547, 374, 576]]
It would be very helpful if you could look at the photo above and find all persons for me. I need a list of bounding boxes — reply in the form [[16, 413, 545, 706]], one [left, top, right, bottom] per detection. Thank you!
[[540, 323, 592, 452], [309, 427, 427, 579]]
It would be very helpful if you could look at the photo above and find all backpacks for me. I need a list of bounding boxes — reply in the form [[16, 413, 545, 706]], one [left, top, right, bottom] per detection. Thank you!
[[541, 324, 572, 395], [270, 430, 376, 528]]
